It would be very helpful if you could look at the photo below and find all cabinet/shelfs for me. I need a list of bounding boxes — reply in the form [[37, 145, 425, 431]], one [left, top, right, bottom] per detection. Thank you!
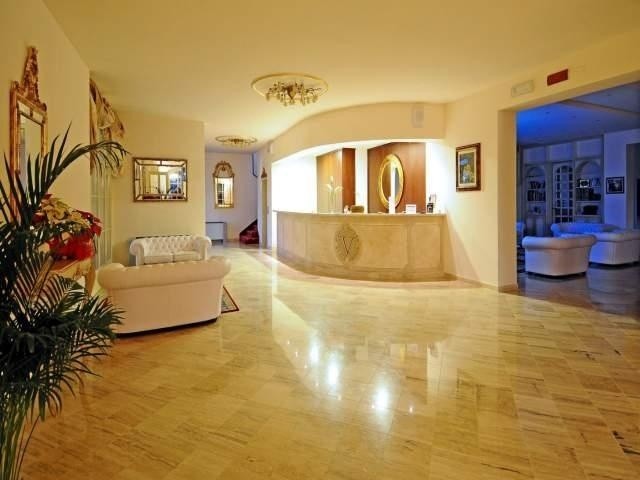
[[316, 148, 355, 212], [525, 172, 601, 234]]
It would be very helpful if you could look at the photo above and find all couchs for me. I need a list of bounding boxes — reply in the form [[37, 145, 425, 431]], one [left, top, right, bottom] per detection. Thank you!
[[97, 255, 232, 334], [130, 235, 212, 266]]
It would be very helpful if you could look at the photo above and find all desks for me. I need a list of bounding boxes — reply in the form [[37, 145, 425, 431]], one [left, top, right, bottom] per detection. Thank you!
[[205, 222, 225, 243], [15, 258, 96, 308]]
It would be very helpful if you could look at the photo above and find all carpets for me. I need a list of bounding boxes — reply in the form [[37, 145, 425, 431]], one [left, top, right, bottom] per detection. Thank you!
[[221, 285, 239, 313]]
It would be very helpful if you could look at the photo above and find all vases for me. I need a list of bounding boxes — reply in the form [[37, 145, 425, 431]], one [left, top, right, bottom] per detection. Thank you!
[[48, 231, 95, 260]]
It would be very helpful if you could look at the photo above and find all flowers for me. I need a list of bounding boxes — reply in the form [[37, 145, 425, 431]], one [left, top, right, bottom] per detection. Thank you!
[[31, 192, 102, 252]]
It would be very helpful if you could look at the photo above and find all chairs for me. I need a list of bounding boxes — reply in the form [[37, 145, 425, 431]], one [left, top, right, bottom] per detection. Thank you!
[[522, 234, 597, 279], [551, 222, 640, 265]]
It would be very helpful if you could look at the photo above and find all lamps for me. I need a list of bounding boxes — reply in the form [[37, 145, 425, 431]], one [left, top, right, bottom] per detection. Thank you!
[[223, 138, 252, 145], [266, 80, 322, 107]]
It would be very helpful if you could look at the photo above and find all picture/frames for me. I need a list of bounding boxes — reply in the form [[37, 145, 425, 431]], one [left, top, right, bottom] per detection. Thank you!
[[456, 143, 480, 189], [606, 177, 624, 194]]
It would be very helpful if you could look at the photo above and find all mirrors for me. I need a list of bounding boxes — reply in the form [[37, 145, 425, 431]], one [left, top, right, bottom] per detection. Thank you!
[[132, 157, 187, 201], [213, 160, 235, 208], [378, 154, 404, 209], [10, 46, 48, 219]]
